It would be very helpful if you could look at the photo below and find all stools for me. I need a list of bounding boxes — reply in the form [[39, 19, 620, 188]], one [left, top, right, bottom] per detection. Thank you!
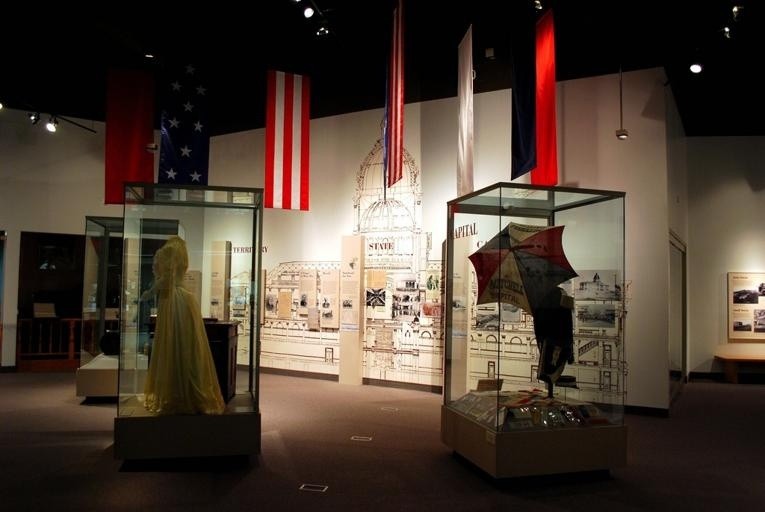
[[714, 351, 765, 385]]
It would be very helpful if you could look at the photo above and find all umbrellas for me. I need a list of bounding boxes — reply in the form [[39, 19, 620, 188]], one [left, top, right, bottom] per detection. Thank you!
[[468, 221, 580, 315]]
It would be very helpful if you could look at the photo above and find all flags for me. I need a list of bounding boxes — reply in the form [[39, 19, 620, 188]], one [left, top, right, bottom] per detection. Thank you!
[[509, 0, 539, 180], [263, 69, 313, 212], [388, 1, 406, 187], [456, 23, 474, 198], [530, 9, 559, 188], [159, 60, 213, 187], [383, 63, 388, 204], [103, 69, 154, 206]]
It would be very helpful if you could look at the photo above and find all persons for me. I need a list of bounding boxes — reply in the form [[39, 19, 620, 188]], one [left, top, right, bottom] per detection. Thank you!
[[266, 296, 275, 312], [300, 294, 307, 306], [140, 235, 227, 416], [322, 297, 330, 308]]
